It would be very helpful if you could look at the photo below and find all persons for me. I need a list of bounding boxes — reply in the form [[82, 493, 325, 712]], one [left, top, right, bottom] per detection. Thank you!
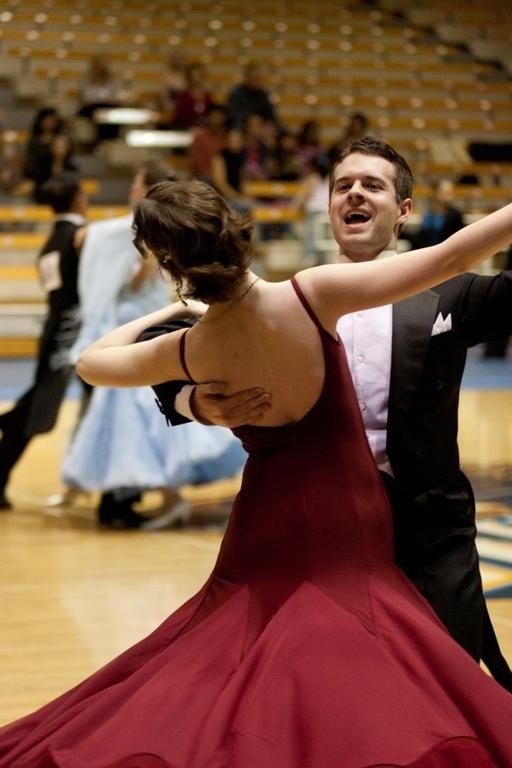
[[1, 52, 465, 532], [135, 137, 512, 692], [0, 179, 512, 768]]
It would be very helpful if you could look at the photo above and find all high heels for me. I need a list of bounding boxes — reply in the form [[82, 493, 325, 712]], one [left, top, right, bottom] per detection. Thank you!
[[46, 481, 192, 532]]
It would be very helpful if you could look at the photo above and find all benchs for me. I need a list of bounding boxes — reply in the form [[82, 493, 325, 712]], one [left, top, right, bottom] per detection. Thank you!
[[0, 1, 512, 363]]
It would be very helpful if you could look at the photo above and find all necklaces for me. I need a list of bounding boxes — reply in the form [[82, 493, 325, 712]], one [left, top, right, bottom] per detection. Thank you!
[[197, 276, 262, 324]]
